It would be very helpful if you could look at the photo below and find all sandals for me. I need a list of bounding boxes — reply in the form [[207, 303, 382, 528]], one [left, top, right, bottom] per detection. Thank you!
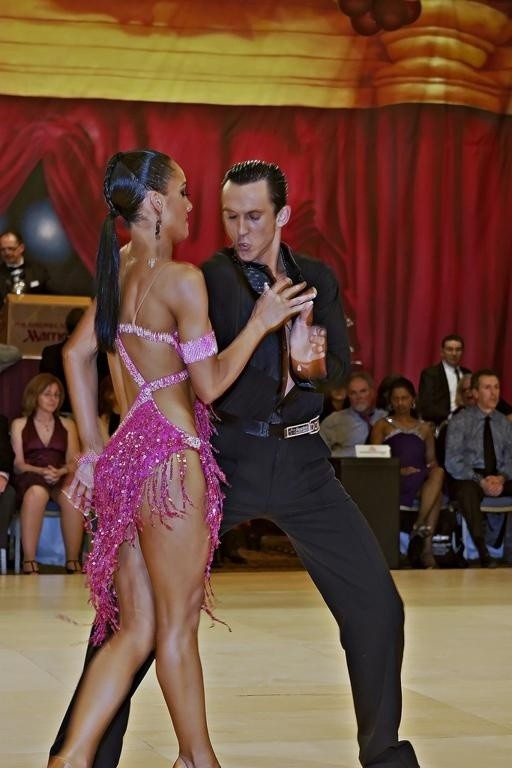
[[65, 560, 83, 574], [21, 559, 42, 577], [406, 533, 437, 569]]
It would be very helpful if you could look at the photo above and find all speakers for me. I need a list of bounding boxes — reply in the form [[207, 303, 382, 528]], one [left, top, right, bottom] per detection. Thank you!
[[334, 455, 403, 571]]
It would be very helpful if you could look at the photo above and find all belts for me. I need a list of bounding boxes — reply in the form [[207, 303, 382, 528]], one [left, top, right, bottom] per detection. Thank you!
[[221, 418, 320, 439]]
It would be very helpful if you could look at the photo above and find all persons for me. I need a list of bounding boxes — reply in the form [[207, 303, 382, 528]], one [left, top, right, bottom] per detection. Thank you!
[[1, 229, 47, 295], [0, 306, 120, 575], [48, 161, 421, 768], [49, 149, 319, 767], [318, 334, 511, 568]]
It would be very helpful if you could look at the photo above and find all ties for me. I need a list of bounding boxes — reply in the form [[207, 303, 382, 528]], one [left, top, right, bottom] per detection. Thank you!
[[482, 415, 501, 477]]
